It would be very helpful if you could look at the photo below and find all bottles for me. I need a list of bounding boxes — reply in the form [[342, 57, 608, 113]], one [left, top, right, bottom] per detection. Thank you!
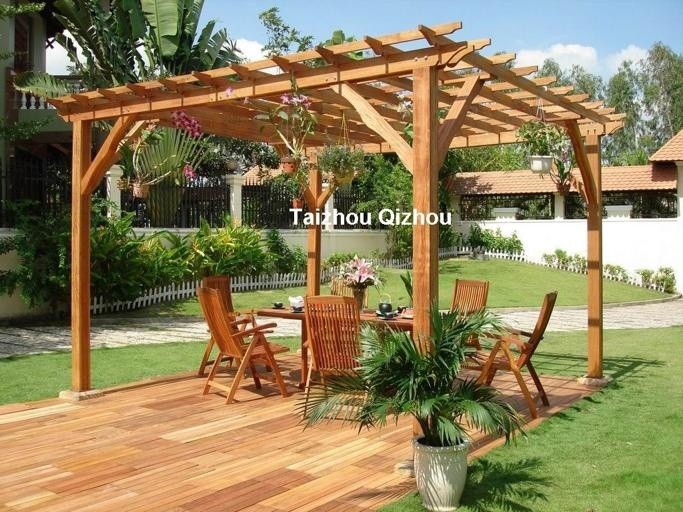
[[379, 302, 392, 315]]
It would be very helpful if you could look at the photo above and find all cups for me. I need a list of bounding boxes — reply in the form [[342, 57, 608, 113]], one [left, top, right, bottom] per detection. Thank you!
[[398, 306, 406, 313], [274, 302, 282, 308], [385, 311, 393, 319], [293, 306, 302, 311]]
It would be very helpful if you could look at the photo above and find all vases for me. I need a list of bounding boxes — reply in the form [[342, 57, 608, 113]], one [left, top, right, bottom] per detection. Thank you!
[[348, 284, 366, 309], [279, 158, 299, 174]]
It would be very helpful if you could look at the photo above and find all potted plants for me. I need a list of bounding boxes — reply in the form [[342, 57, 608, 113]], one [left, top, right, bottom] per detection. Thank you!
[[285, 183, 304, 210], [132, 181, 151, 199], [318, 144, 363, 185], [292, 298, 532, 511], [514, 119, 568, 176], [546, 157, 572, 194]]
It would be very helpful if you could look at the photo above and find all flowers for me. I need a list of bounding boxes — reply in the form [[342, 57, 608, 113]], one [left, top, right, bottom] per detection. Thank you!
[[224, 84, 313, 158], [340, 254, 383, 289], [127, 110, 214, 183]]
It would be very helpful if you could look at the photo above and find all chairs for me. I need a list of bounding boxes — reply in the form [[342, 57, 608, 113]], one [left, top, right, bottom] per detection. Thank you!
[[457, 291, 559, 418], [450, 278, 489, 350], [196, 285, 289, 405], [199, 274, 270, 376], [302, 293, 370, 421]]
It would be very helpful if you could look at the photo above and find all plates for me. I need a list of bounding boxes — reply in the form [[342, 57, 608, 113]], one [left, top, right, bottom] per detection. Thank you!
[[401, 315, 413, 319], [375, 309, 399, 316], [273, 307, 285, 309], [292, 311, 304, 313]]
